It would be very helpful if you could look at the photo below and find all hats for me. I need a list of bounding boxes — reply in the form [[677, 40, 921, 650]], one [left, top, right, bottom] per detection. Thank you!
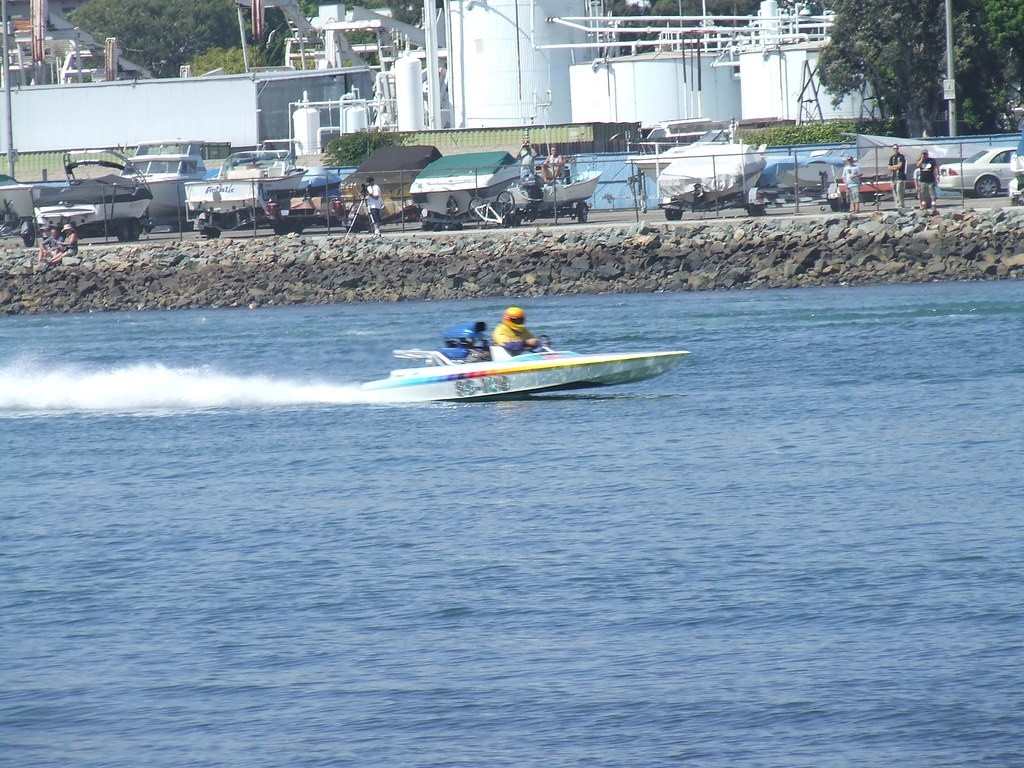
[[848, 157, 853, 161], [61, 224, 73, 232], [922, 150, 928, 154]]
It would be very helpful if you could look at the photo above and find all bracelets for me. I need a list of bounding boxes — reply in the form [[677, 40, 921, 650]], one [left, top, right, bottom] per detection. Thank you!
[[937, 174, 940, 175]]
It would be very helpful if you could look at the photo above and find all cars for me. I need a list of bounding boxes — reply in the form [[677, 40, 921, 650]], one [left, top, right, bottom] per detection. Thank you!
[[937, 146, 1020, 198]]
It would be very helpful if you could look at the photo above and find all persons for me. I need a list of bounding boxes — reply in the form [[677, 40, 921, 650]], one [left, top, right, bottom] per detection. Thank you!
[[888, 144, 906, 207], [446, 195, 460, 216], [913, 149, 940, 210], [541, 146, 563, 183], [367, 177, 382, 239], [39, 224, 77, 266], [516, 138, 537, 180], [842, 157, 863, 212], [493, 307, 540, 356]]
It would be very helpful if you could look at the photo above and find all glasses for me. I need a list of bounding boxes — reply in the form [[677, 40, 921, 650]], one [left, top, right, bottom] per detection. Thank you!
[[894, 148, 898, 150]]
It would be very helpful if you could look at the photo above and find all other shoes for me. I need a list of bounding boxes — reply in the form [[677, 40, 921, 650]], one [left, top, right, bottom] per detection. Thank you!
[[920, 204, 926, 210], [893, 208, 898, 211], [852, 210, 859, 214], [931, 205, 936, 210]]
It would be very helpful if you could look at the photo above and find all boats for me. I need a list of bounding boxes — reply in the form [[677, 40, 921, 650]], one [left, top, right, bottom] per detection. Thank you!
[[409, 150, 524, 217], [339, 145, 444, 224], [508, 171, 606, 209], [756, 145, 937, 197], [357, 349, 694, 402], [0, 150, 153, 236], [624, 144, 767, 208], [118, 139, 207, 226], [185, 150, 360, 219]]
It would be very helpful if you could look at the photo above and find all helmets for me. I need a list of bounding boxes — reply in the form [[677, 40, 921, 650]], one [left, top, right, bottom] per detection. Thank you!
[[503, 307, 524, 332]]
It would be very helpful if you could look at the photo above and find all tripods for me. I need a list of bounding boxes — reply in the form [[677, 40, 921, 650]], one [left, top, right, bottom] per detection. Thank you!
[[344, 198, 383, 242]]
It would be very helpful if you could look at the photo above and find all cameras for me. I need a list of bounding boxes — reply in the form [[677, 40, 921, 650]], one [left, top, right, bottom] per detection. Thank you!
[[361, 184, 367, 193]]
